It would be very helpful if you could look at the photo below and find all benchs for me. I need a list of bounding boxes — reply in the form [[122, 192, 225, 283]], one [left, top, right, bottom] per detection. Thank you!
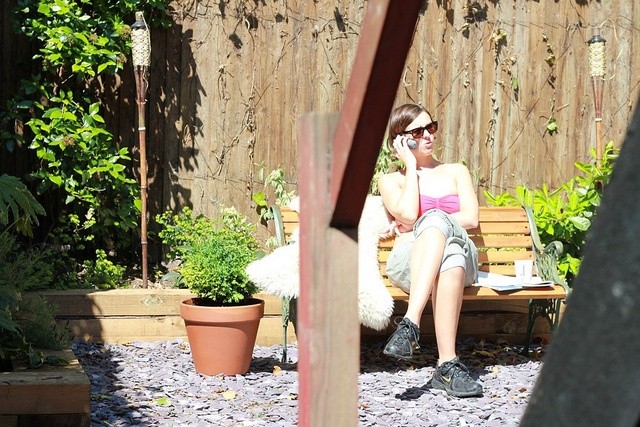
[[261, 202, 569, 360]]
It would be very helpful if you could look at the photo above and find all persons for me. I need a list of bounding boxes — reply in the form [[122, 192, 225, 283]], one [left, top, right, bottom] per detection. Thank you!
[[377, 104, 483, 396]]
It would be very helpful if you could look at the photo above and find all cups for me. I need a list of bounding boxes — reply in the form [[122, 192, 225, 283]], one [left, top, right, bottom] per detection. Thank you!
[[514, 260, 533, 288], [534, 251, 558, 287]]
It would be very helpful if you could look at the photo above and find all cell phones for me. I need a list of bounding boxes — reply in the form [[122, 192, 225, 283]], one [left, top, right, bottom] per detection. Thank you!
[[401, 138, 417, 148]]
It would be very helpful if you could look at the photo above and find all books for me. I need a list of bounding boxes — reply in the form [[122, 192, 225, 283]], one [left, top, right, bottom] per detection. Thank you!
[[476, 260, 554, 292]]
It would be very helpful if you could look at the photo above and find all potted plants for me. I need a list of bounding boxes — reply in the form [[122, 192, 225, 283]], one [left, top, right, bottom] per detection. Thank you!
[[0, 172, 94, 427], [153, 206, 266, 378]]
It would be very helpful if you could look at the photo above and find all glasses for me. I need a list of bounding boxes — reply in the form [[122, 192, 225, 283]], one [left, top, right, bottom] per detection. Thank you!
[[401, 120, 438, 139]]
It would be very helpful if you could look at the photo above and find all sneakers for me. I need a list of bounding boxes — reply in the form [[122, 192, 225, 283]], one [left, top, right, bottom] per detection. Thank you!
[[430, 356, 483, 398], [383, 317, 421, 361]]
